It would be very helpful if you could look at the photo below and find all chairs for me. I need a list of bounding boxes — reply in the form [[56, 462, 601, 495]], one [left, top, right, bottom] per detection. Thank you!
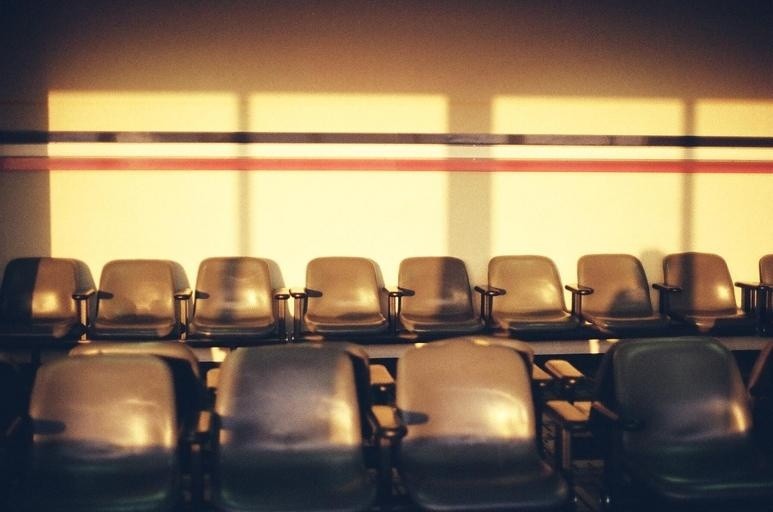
[[566, 254, 682, 335], [174, 256, 290, 343], [484, 254, 595, 338], [653, 252, 765, 336], [398, 255, 495, 339], [73, 259, 191, 339], [291, 256, 403, 342], [737, 254, 772, 321], [1, 256, 95, 364]]
